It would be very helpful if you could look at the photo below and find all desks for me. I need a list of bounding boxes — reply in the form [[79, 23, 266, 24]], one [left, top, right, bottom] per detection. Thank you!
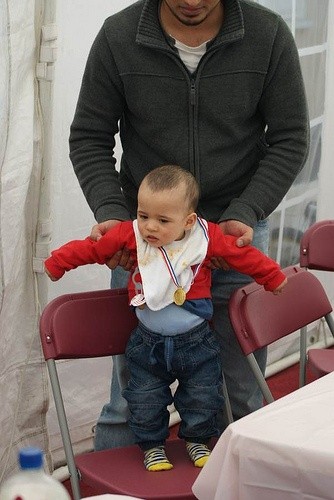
[[191, 367, 334, 500]]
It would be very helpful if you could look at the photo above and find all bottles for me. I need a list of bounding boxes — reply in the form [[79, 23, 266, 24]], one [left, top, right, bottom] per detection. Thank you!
[[0, 445, 72, 500]]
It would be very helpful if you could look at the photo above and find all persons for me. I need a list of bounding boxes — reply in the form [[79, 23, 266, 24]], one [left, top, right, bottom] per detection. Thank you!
[[69, 0, 311, 451], [44, 165, 289, 471]]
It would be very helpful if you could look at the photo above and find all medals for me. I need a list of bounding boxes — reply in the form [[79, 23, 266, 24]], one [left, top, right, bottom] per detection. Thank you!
[[152, 216, 210, 306]]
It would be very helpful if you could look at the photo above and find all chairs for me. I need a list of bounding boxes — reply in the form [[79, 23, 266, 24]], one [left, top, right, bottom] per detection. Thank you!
[[40, 220, 334, 500]]
